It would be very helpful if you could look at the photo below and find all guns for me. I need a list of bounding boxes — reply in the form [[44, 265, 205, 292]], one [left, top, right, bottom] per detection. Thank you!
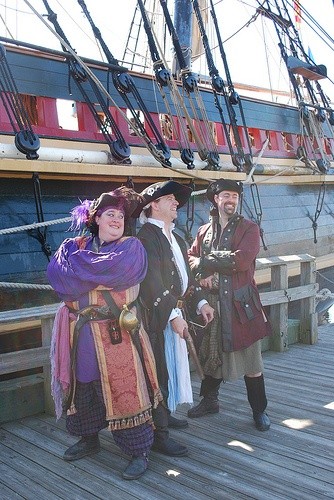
[[183, 328, 205, 380]]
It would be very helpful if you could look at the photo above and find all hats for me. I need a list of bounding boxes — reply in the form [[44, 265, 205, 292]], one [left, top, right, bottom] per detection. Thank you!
[[206, 180, 243, 206], [131, 180, 193, 220], [93, 193, 130, 220]]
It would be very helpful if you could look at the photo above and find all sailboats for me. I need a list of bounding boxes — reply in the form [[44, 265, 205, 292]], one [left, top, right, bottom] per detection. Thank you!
[[0, 0, 334, 379]]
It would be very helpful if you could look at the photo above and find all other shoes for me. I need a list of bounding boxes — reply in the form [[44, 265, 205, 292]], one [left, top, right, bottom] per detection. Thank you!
[[168, 415, 189, 429], [252, 409, 271, 431], [187, 397, 220, 418], [63, 436, 102, 460], [152, 430, 189, 457], [121, 452, 151, 480]]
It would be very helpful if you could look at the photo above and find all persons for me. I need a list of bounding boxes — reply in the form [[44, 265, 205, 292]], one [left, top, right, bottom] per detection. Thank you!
[[136, 180, 215, 457], [187, 179, 271, 432], [47, 195, 154, 479]]
